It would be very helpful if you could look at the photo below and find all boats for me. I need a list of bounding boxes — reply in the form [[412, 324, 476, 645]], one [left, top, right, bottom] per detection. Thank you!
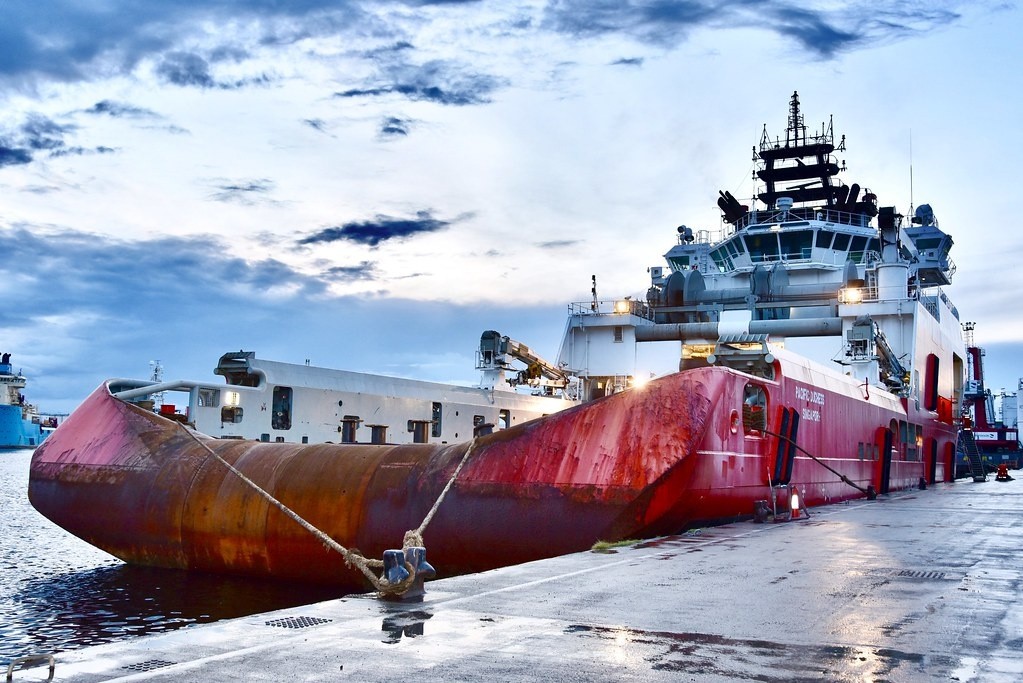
[[21, 89, 966, 585], [0, 352, 61, 456], [952, 318, 1023, 477]]
